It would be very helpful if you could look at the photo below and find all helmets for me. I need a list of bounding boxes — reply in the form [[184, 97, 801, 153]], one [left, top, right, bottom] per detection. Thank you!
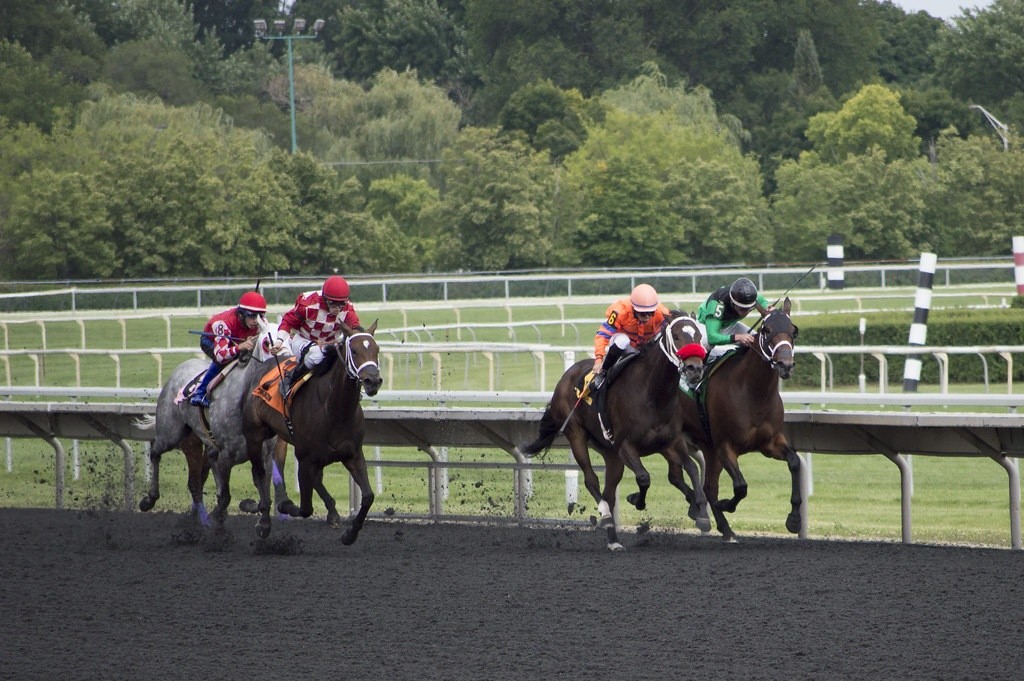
[[238, 291, 267, 311], [323, 276, 350, 301], [630, 283, 659, 312], [729, 277, 758, 308]]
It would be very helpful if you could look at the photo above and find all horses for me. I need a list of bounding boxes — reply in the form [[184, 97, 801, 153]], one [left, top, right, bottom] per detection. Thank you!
[[138, 315, 384, 549], [520, 297, 805, 557]]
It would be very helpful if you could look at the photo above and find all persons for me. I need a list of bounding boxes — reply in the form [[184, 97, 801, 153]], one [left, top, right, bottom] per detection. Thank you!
[[190, 290, 268, 408], [270, 276, 362, 396], [695, 277, 777, 365], [592, 282, 673, 374]]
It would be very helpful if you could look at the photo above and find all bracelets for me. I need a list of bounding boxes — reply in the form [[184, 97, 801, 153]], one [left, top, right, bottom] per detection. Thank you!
[[277, 338, 283, 342]]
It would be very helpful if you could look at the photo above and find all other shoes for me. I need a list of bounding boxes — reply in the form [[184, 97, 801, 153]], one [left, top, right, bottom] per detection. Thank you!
[[589, 368, 608, 391]]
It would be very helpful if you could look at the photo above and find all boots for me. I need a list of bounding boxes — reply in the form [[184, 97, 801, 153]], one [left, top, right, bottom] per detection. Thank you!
[[279, 361, 311, 398], [190, 361, 221, 407]]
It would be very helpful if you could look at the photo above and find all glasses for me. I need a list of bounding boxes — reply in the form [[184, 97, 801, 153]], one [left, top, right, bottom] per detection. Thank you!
[[638, 312, 654, 317], [325, 300, 346, 307], [740, 305, 757, 311], [245, 310, 264, 318]]
